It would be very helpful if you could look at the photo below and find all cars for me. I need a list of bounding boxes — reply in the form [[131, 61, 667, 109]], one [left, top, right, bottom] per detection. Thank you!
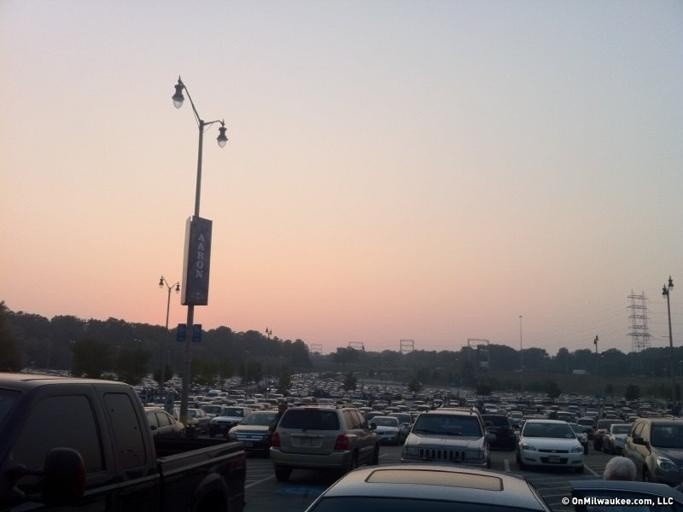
[[512, 418, 585, 473], [568, 480, 682, 512], [304, 461, 552, 511], [620, 417, 683, 488]]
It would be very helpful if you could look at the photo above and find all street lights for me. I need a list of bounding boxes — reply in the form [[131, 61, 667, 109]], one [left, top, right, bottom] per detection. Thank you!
[[517, 312, 524, 367], [593, 334, 598, 397], [661, 275, 676, 415], [170, 76, 227, 424], [264, 326, 272, 390], [158, 275, 180, 403]]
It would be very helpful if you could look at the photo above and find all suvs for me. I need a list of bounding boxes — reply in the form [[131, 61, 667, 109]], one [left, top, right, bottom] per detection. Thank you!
[[269, 401, 379, 482], [399, 405, 496, 469]]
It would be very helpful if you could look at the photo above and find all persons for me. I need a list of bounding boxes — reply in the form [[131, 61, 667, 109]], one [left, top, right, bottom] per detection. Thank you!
[[603, 455, 638, 481]]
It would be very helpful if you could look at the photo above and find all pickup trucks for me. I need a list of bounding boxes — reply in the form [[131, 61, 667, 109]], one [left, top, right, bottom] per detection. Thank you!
[[0, 369, 246, 511]]
